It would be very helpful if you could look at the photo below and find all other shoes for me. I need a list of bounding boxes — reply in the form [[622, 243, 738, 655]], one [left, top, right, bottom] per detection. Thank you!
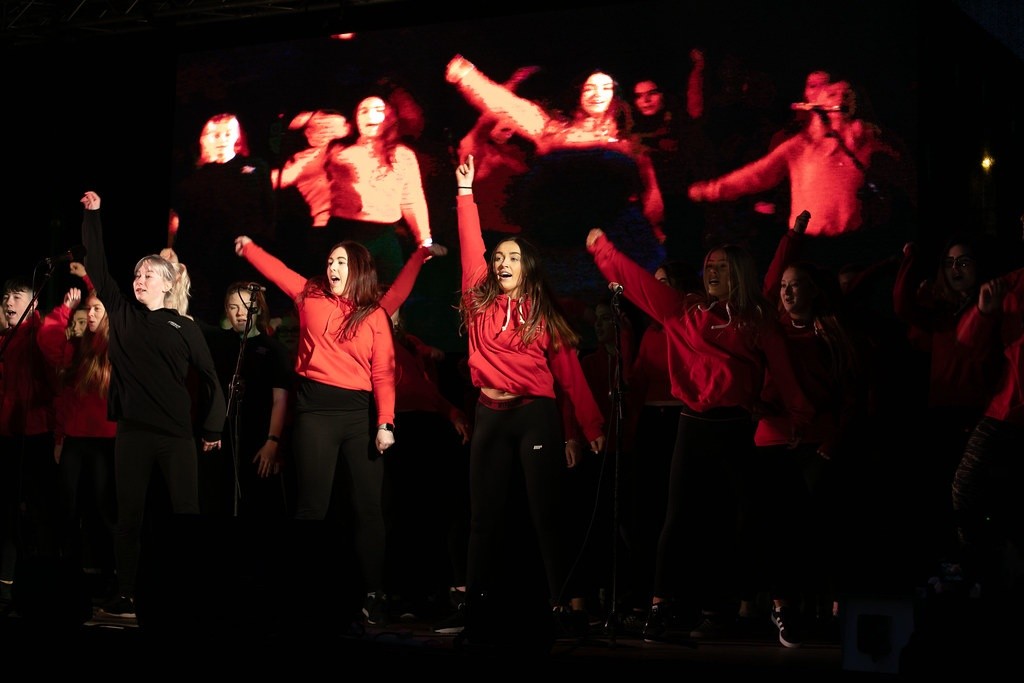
[[642, 601, 666, 644], [357, 585, 641, 634], [769, 605, 800, 649]]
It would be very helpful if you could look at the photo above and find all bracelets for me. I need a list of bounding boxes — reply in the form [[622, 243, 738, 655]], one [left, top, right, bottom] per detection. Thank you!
[[565, 439, 579, 444]]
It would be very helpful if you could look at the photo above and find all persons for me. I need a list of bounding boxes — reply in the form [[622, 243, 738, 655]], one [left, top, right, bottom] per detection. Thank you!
[[0, 45, 1024, 649]]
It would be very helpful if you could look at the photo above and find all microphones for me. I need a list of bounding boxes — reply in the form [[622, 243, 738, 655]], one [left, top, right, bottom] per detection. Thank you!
[[609, 281, 624, 295], [792, 103, 849, 114], [40, 245, 88, 265], [241, 285, 266, 292]]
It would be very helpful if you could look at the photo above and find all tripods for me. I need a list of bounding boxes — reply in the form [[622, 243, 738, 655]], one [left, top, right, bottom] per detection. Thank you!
[[557, 297, 701, 653]]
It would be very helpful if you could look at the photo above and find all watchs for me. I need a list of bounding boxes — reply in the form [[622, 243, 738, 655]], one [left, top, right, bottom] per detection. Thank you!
[[378, 422, 394, 433], [267, 435, 280, 443]]
